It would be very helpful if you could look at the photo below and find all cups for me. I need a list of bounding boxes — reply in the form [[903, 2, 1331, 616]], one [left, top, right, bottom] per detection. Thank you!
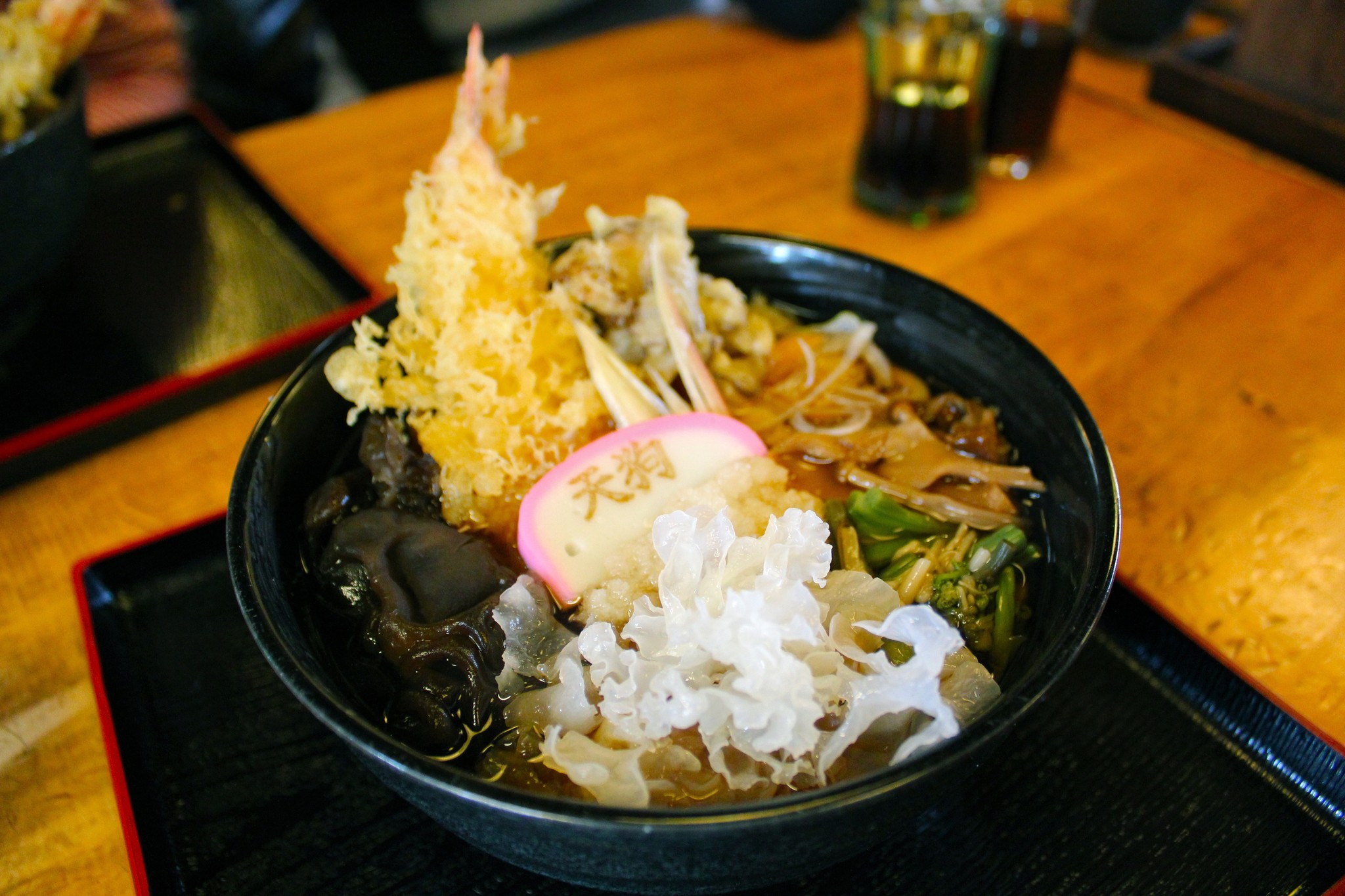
[[978, 0, 1083, 178], [852, 2, 1002, 228]]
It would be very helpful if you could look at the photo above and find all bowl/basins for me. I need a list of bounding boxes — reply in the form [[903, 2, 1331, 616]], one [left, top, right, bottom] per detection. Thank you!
[[1, 64, 94, 286], [222, 229, 1119, 891]]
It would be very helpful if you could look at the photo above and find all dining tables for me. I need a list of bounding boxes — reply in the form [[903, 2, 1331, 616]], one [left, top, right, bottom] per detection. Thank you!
[[3, 25, 1345, 895]]
